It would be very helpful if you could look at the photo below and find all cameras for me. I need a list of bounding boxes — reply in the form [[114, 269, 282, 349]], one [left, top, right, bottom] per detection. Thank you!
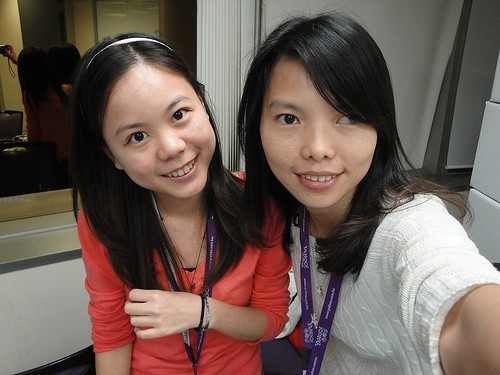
[[0, 45, 10, 54]]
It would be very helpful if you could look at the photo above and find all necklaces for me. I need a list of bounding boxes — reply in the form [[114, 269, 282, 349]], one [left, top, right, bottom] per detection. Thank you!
[[144, 182, 219, 375], [297, 204, 346, 375]]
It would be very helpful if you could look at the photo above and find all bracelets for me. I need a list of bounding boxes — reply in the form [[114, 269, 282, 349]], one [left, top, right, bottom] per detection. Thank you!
[[195, 293, 211, 331]]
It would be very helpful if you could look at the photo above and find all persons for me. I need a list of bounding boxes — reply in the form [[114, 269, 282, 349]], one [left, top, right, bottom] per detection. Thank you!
[[70, 32, 294, 375], [0, 41, 82, 163], [236, 12, 500, 375]]
